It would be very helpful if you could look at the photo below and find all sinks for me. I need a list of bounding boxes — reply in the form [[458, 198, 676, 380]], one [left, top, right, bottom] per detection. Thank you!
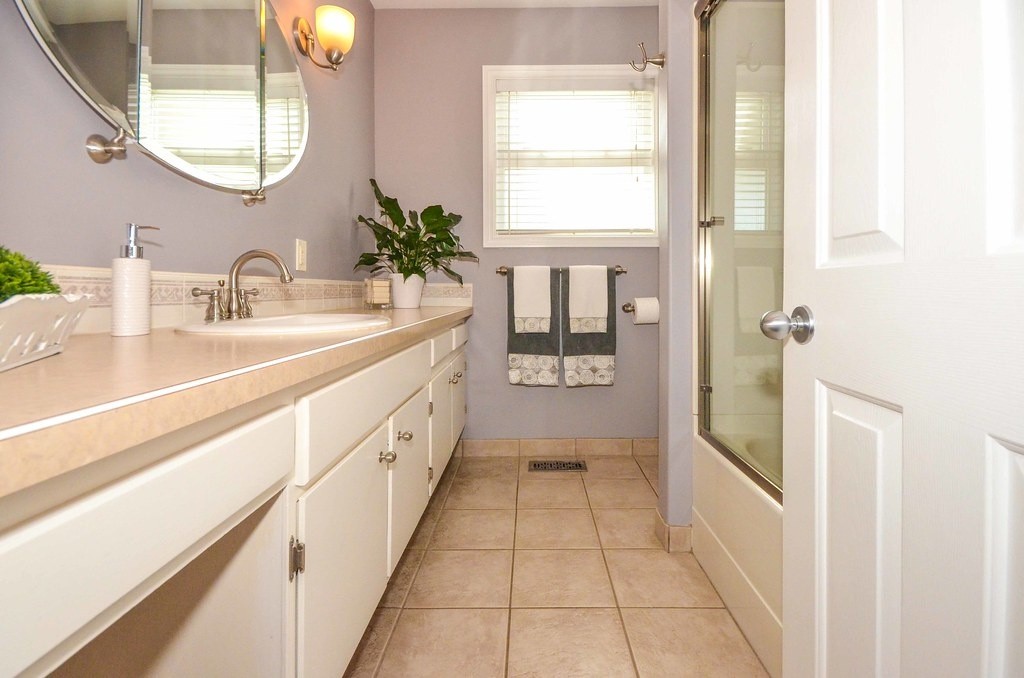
[[174, 312, 393, 334]]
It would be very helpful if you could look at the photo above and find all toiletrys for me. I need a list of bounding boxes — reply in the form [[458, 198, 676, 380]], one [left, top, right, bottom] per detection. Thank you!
[[110, 222, 161, 337]]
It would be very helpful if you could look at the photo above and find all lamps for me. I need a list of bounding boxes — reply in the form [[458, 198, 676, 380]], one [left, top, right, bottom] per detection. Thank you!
[[293, 5, 354, 71]]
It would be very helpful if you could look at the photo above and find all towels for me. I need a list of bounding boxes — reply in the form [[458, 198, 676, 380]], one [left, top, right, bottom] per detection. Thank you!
[[506, 265, 616, 388]]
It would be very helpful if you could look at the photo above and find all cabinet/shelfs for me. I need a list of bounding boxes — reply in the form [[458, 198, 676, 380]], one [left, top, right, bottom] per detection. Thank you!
[[0, 344, 433, 678], [428, 322, 467, 498]]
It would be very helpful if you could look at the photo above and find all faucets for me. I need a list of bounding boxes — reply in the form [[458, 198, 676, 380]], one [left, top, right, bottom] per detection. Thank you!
[[227, 248, 294, 321]]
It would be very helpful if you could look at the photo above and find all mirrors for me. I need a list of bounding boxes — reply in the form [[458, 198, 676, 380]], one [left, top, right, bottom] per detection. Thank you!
[[17, 1, 310, 205]]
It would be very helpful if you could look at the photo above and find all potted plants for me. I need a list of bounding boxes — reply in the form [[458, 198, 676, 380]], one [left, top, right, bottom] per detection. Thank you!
[[352, 178, 480, 308], [0, 243, 89, 378]]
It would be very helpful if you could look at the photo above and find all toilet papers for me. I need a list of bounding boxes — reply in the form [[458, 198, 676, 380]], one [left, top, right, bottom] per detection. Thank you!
[[630, 297, 661, 325]]
